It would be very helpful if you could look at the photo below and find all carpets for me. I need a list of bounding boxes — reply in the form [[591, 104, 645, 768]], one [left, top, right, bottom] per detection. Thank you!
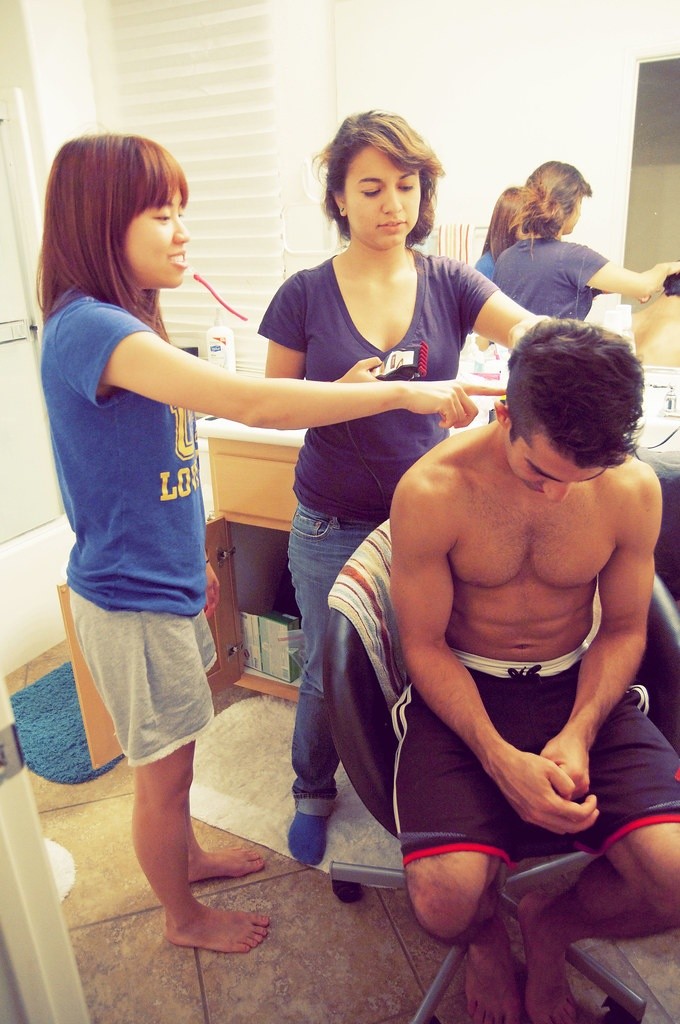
[[188, 695, 404, 889], [11, 662, 124, 785]]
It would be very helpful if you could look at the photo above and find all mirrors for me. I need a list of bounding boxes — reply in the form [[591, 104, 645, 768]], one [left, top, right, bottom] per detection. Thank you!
[[281, 0, 680, 371]]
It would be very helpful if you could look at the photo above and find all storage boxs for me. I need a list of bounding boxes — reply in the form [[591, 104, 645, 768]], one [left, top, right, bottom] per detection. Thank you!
[[238, 609, 303, 683]]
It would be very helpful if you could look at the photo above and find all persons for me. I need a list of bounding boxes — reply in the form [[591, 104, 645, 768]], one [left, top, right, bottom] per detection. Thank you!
[[492, 161, 680, 322], [39, 134, 510, 952], [389, 320, 680, 1024], [258, 112, 550, 863], [474, 187, 522, 282]]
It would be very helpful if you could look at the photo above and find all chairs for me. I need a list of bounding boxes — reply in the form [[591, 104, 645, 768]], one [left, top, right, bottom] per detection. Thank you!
[[324, 518, 680, 1024]]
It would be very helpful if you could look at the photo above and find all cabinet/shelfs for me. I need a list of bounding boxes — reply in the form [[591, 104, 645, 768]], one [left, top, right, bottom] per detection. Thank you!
[[55, 413, 307, 769]]
